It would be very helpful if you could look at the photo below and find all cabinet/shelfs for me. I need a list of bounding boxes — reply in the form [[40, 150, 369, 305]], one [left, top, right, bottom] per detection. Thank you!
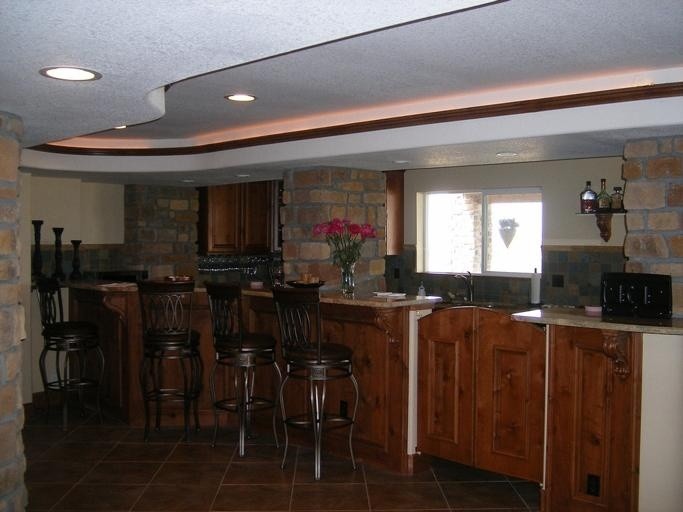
[[198, 181, 272, 256], [545, 326, 682, 512], [417, 304, 545, 485]]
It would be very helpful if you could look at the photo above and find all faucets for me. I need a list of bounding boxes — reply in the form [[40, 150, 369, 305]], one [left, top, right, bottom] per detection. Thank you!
[[453, 271, 474, 302]]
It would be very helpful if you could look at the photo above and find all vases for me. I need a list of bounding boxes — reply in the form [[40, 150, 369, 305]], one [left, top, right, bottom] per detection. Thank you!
[[341, 260, 356, 299]]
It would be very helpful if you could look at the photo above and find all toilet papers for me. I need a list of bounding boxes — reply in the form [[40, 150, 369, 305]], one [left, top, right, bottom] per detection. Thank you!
[[531, 273, 541, 304]]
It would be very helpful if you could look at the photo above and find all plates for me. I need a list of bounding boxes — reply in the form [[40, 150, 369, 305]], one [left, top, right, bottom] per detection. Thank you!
[[584, 306, 602, 312], [285, 281, 324, 288]]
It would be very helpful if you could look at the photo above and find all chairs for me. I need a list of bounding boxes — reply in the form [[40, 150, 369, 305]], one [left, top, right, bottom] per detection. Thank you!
[[37, 276, 105, 432], [270, 284, 360, 482], [134, 277, 204, 444], [205, 281, 283, 458]]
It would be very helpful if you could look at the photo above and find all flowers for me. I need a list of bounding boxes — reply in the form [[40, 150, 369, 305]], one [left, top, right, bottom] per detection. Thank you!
[[313, 217, 378, 293]]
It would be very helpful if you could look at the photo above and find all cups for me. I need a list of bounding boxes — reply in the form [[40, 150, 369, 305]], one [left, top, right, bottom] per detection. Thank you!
[[250, 281, 264, 290]]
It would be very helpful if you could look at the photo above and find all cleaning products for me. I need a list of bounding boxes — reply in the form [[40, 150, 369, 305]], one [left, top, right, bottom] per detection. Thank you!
[[418, 282, 426, 296]]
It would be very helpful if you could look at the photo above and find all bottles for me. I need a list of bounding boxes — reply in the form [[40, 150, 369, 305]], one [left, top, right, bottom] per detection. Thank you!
[[418, 281, 425, 296], [580, 179, 625, 214], [165, 275, 193, 282]]
